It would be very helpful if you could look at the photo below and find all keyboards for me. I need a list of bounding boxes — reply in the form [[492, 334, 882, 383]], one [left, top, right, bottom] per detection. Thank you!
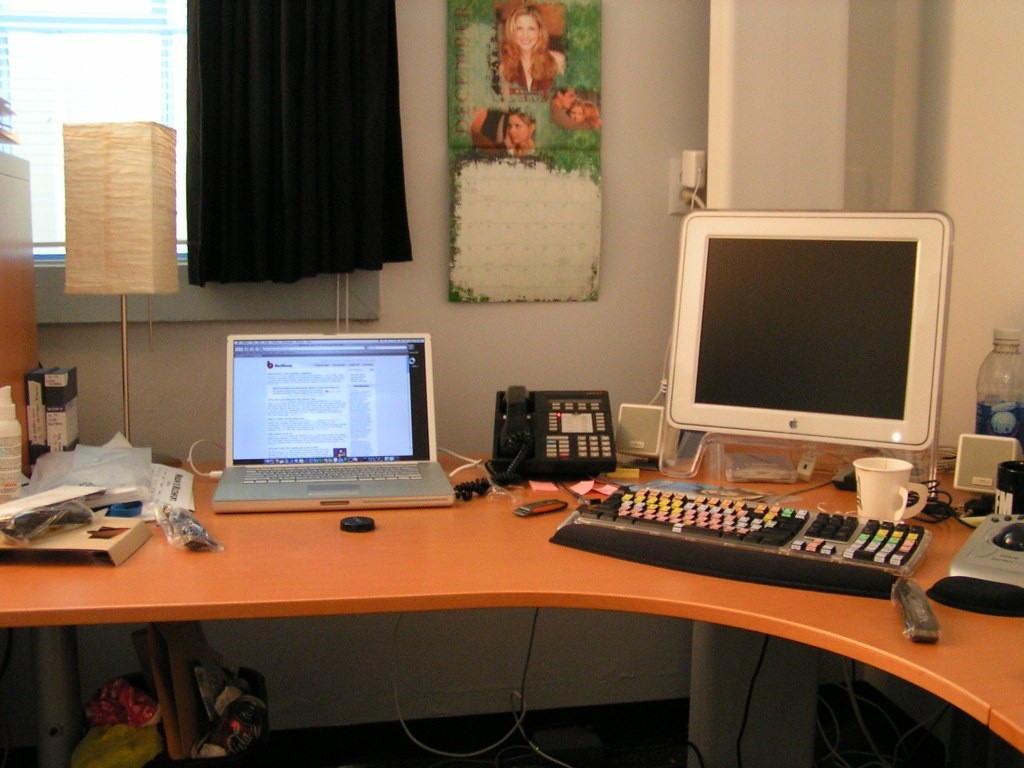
[[548, 485, 932, 601]]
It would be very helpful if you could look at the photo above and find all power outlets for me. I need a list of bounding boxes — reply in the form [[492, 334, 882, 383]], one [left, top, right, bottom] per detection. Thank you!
[[668, 166, 696, 216]]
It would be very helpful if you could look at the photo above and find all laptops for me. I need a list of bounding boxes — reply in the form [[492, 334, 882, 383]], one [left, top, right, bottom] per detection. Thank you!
[[210, 333, 456, 514]]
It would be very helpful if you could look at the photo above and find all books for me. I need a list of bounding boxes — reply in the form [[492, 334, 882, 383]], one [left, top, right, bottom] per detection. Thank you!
[[23, 366, 80, 476]]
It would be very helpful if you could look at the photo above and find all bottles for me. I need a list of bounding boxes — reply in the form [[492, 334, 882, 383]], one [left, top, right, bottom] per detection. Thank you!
[[0, 385, 22, 495], [975, 328, 1024, 453]]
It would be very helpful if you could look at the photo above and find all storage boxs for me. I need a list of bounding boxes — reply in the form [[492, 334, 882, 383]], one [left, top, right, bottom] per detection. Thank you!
[[726, 452, 796, 483]]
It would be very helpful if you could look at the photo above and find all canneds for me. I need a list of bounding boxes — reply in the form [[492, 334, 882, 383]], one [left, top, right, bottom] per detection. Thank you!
[[199, 695, 269, 756]]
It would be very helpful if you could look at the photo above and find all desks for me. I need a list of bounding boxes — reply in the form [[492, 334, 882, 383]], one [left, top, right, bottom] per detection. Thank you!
[[0, 456, 1024, 768]]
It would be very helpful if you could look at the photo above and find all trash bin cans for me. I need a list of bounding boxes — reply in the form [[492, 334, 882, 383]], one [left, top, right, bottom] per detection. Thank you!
[[83, 663, 269, 768]]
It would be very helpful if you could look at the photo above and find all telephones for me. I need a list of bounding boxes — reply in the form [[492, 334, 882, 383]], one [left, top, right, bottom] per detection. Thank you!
[[489, 388, 617, 478]]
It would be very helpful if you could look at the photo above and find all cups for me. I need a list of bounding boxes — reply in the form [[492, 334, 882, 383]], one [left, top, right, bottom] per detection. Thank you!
[[995, 461, 1024, 515], [853, 457, 929, 523]]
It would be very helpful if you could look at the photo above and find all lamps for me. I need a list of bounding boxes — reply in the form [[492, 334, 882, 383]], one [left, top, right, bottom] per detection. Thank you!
[[62, 120, 186, 469]]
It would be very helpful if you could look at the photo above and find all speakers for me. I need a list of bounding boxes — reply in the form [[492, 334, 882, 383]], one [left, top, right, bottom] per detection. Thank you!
[[614, 404, 666, 469], [953, 433, 1023, 529]]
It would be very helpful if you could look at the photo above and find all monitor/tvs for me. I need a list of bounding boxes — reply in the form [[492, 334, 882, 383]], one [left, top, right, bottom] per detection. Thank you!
[[657, 209, 956, 497]]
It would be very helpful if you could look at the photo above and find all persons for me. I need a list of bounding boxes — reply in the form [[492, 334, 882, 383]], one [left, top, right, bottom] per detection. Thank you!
[[499, 6, 601, 156]]
